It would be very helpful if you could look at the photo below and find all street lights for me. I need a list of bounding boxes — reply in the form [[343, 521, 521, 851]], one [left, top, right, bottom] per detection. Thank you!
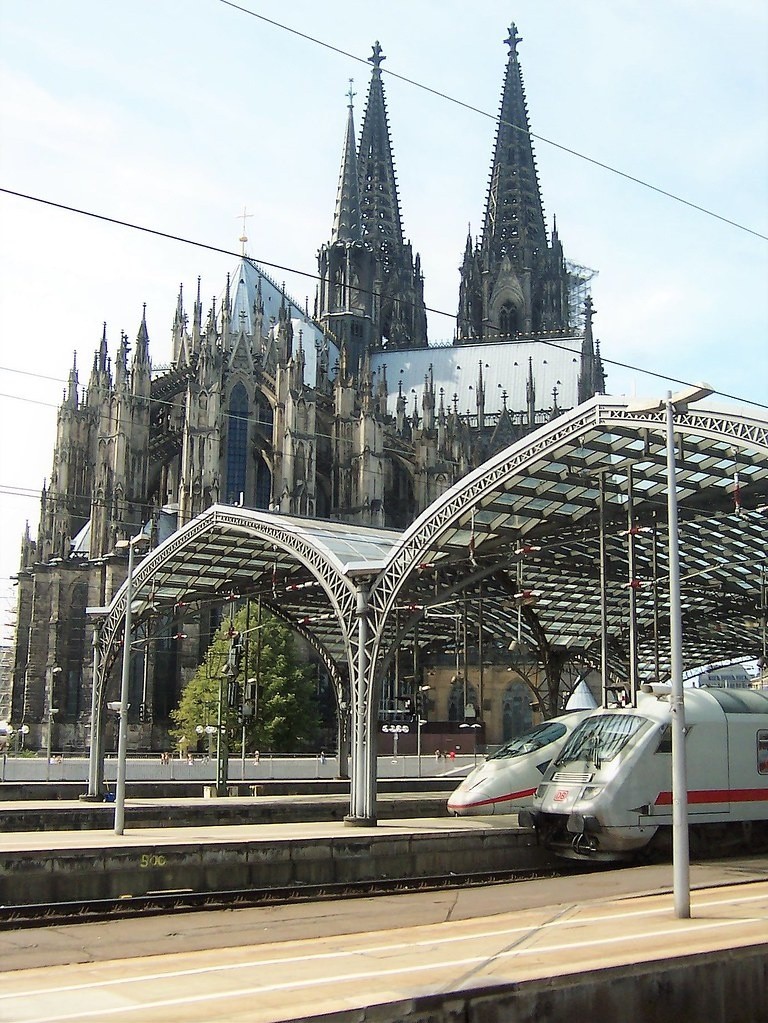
[[112, 534, 152, 834]]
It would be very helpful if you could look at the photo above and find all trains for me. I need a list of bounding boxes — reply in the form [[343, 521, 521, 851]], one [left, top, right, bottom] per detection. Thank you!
[[514, 681, 768, 871], [444, 708, 600, 816]]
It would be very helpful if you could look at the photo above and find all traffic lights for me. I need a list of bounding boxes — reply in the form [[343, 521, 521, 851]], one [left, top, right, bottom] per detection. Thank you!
[[416, 692, 424, 715], [394, 694, 414, 716], [227, 681, 243, 708], [229, 647, 246, 670]]
